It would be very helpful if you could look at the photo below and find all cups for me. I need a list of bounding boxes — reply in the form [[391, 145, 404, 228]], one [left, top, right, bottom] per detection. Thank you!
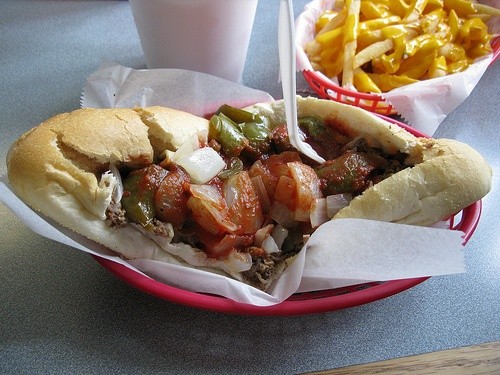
[[129, 0, 255, 88]]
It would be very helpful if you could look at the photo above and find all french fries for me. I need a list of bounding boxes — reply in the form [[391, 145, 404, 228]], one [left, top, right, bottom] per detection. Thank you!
[[304, 1, 500, 95]]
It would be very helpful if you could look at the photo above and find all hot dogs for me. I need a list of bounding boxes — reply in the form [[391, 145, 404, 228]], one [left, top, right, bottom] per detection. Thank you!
[[6, 95, 492, 290]]
